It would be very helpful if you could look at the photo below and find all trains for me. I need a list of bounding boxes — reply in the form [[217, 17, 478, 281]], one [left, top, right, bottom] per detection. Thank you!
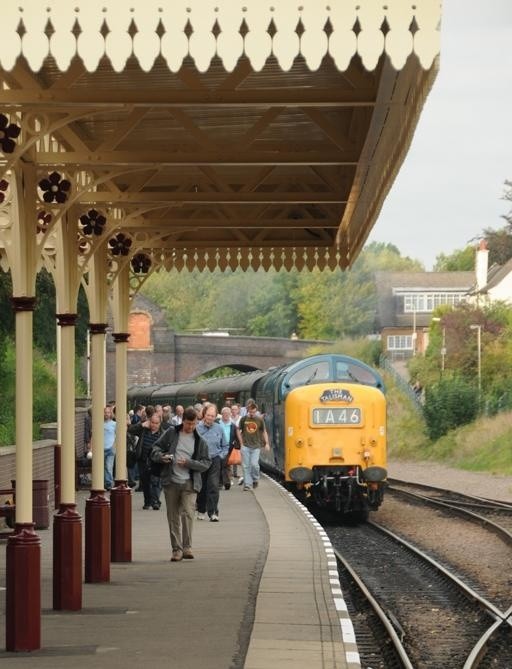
[[127, 353, 390, 520]]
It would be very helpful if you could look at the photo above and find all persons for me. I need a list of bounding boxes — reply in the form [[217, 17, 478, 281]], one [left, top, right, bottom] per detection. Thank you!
[[84, 391, 271, 525], [150, 408, 213, 562]]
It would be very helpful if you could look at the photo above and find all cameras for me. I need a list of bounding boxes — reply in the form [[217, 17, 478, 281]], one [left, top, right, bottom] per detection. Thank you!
[[166, 454, 174, 461]]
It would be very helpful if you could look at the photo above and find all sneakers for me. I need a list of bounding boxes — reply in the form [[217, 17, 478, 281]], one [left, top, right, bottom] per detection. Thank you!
[[224, 481, 231, 491], [143, 505, 150, 509], [183, 546, 194, 559], [238, 477, 245, 485], [210, 514, 218, 521], [171, 549, 183, 561], [243, 487, 249, 491], [153, 504, 159, 510], [253, 481, 258, 489], [197, 512, 207, 520]]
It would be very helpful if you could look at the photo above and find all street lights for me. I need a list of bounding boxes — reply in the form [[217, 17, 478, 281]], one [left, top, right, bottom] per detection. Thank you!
[[432, 318, 445, 371], [470, 325, 481, 411]]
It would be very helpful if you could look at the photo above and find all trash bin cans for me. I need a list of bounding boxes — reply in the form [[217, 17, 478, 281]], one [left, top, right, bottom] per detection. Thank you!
[[10, 479, 49, 530]]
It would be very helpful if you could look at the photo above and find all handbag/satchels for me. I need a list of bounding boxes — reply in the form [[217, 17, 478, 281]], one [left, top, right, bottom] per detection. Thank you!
[[147, 447, 169, 476], [227, 446, 242, 466], [133, 429, 145, 461]]
